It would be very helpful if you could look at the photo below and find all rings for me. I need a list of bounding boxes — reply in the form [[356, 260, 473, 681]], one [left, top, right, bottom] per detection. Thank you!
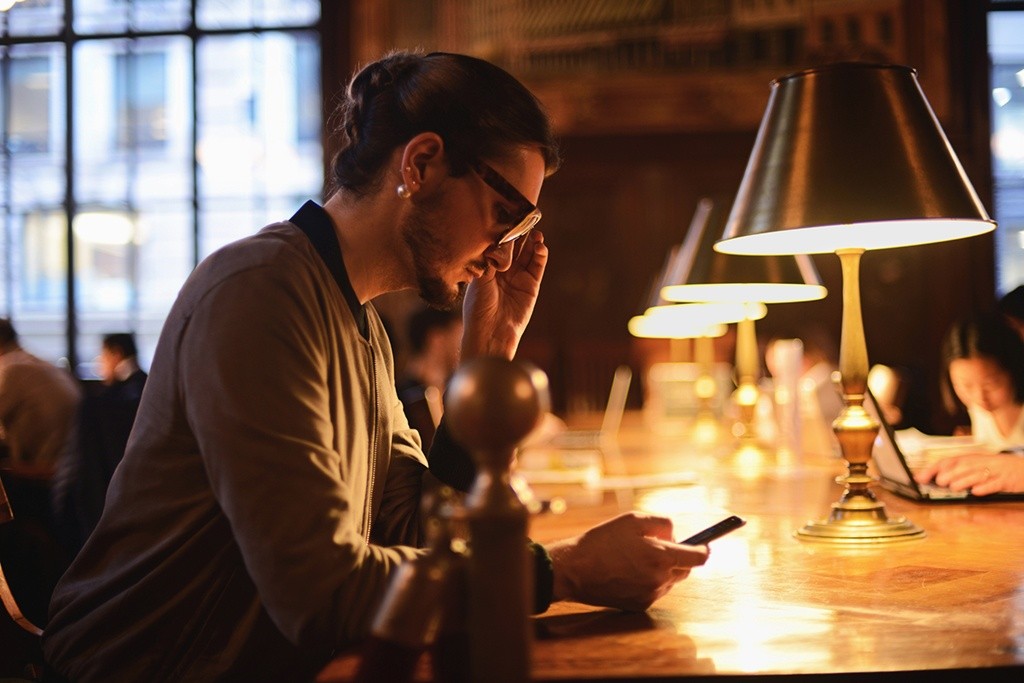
[[984, 467, 992, 476]]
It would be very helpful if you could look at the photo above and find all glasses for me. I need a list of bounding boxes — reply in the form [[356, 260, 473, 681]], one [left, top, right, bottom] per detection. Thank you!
[[443, 137, 542, 244]]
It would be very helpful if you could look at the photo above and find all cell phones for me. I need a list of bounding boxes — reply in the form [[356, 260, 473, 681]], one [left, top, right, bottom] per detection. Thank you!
[[682, 515, 747, 545]]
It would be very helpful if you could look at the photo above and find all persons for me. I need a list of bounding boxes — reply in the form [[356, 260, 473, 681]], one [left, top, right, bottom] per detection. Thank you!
[[42, 48, 711, 682], [0, 317, 85, 509], [77, 331, 149, 483], [915, 280, 1024, 494], [387, 279, 467, 459]]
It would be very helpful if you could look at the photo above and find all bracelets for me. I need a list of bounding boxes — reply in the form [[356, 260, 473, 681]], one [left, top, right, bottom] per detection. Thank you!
[[528, 538, 552, 615]]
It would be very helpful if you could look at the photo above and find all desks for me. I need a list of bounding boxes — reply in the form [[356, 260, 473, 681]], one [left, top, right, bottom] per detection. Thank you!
[[314, 407, 1024, 683]]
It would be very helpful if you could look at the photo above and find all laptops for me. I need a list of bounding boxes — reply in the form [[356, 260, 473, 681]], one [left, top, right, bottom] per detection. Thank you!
[[816, 377, 1024, 505]]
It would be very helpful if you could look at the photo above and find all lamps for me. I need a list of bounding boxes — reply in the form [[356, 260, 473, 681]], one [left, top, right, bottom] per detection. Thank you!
[[627, 62, 999, 545]]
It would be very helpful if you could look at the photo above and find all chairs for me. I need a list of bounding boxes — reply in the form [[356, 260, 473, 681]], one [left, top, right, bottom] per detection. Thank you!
[[0, 467, 83, 638]]
